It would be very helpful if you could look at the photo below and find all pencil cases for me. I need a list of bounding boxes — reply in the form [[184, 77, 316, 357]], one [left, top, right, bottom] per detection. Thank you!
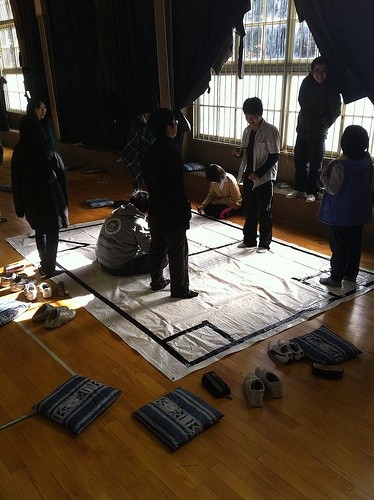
[[202, 371, 231, 398], [312, 362, 344, 379]]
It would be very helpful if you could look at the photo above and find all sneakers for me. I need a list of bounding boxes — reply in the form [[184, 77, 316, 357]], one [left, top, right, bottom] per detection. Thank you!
[[255, 366, 283, 397], [24, 283, 38, 301], [305, 194, 316, 202], [1, 272, 28, 287], [268, 341, 293, 364], [286, 190, 306, 199], [277, 339, 304, 361], [39, 282, 53, 298], [10, 275, 37, 292], [243, 372, 265, 407]]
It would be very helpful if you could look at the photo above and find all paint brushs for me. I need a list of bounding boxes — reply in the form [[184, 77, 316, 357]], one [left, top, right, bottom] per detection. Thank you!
[[195, 204, 202, 215]]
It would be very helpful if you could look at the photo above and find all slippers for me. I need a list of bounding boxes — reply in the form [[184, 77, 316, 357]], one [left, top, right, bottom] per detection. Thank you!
[[45, 309, 76, 329], [32, 304, 69, 322]]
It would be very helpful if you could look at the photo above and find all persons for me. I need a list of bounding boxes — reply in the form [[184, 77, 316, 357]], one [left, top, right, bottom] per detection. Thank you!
[[96, 190, 169, 277], [232, 98, 281, 253], [11, 113, 68, 277], [286, 58, 341, 202], [197, 164, 242, 219], [143, 107, 198, 298], [115, 96, 159, 190], [315, 125, 374, 287], [27, 94, 57, 149], [0, 70, 9, 222]]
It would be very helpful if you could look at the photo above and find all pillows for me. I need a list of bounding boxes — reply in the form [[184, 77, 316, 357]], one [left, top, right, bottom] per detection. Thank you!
[[184, 162, 205, 172], [290, 325, 363, 366], [84, 196, 114, 209], [31, 375, 122, 437], [0, 184, 13, 192], [131, 386, 224, 452]]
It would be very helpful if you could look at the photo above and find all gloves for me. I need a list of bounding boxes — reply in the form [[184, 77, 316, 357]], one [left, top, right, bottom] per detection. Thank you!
[[201, 371, 232, 400]]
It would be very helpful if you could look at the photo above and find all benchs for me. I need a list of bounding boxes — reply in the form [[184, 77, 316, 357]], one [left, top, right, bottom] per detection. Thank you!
[[186, 170, 374, 247]]
